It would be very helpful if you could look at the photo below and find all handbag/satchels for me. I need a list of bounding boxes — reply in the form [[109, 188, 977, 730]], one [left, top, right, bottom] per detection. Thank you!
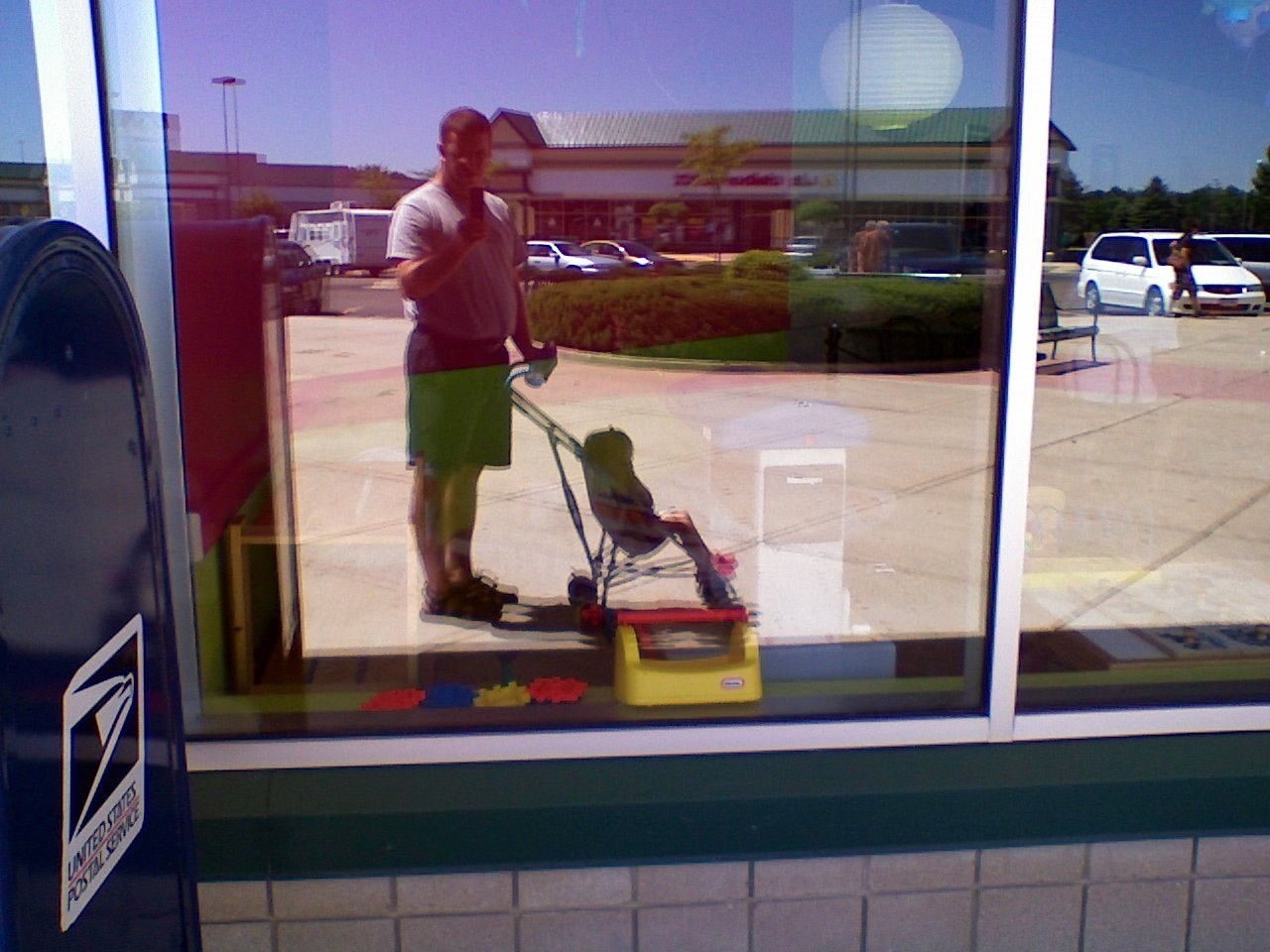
[[1167, 252, 1185, 269]]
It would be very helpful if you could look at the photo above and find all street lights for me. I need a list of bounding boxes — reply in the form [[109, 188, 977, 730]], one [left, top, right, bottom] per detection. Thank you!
[[210, 73, 247, 219], [956, 124, 988, 275]]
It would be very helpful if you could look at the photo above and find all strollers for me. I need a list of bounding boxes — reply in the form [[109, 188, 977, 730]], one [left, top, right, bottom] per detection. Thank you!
[[502, 359, 748, 634]]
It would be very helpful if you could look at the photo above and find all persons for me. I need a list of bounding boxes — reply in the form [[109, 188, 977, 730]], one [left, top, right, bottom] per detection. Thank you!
[[583, 429, 736, 608], [846, 221, 891, 273], [386, 109, 555, 620], [1163, 231, 1209, 317]]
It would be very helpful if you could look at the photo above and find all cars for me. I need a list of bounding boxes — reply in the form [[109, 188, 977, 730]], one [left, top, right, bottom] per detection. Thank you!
[[1076, 229, 1267, 317], [781, 236, 822, 257], [580, 239, 682, 268], [1208, 234, 1270, 302], [525, 240, 627, 274], [273, 238, 322, 314]]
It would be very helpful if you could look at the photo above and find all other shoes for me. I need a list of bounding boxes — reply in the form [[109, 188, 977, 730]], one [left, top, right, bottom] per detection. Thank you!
[[422, 587, 503, 620], [1194, 310, 1209, 316], [446, 574, 518, 604], [710, 553, 739, 579]]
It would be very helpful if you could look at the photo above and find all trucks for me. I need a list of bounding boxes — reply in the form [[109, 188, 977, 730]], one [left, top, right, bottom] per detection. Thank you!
[[286, 201, 394, 279]]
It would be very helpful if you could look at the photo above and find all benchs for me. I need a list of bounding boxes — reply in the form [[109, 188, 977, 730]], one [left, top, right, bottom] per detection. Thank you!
[[1037, 283, 1099, 364]]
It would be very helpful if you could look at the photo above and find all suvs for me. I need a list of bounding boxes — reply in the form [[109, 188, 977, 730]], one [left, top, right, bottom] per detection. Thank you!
[[885, 221, 986, 275]]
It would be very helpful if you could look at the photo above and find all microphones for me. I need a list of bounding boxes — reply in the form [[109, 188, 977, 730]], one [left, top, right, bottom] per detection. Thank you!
[[470, 187, 484, 222]]
